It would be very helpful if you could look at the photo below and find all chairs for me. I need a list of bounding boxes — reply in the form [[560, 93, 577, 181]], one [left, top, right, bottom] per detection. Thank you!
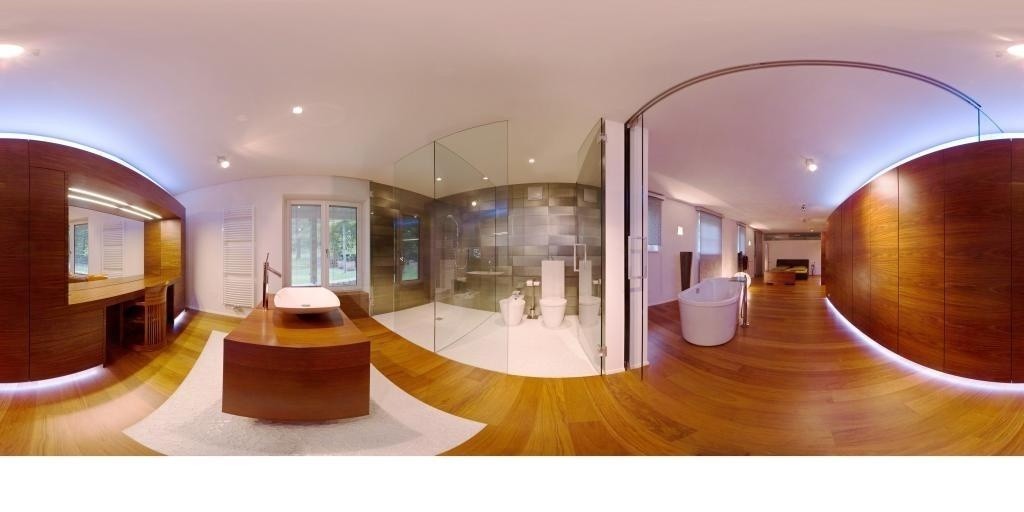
[[119, 281, 168, 354]]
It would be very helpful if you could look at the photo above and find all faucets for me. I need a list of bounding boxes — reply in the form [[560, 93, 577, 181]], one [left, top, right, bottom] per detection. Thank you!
[[263, 252, 282, 310], [487, 260, 493, 272], [728, 275, 747, 283]]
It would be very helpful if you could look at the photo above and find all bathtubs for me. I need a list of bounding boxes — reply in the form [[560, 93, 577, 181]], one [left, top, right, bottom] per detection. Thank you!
[[677, 276, 743, 347]]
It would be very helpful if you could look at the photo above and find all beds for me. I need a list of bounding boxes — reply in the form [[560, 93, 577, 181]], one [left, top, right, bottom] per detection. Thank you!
[[770, 259, 809, 280]]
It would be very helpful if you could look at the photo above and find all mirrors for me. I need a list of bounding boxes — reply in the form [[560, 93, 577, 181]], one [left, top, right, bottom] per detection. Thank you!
[[68, 206, 145, 281]]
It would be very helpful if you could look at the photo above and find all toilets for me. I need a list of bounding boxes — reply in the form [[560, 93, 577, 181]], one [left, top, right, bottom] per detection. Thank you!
[[579, 260, 601, 327], [539, 260, 568, 331]]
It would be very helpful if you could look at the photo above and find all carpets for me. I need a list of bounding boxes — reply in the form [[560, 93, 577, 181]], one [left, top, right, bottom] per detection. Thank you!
[[123, 327, 488, 454]]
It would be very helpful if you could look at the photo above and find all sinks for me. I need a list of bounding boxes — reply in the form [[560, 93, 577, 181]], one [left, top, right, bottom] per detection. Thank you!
[[499, 294, 526, 326], [465, 270, 504, 279], [272, 286, 340, 315]]
